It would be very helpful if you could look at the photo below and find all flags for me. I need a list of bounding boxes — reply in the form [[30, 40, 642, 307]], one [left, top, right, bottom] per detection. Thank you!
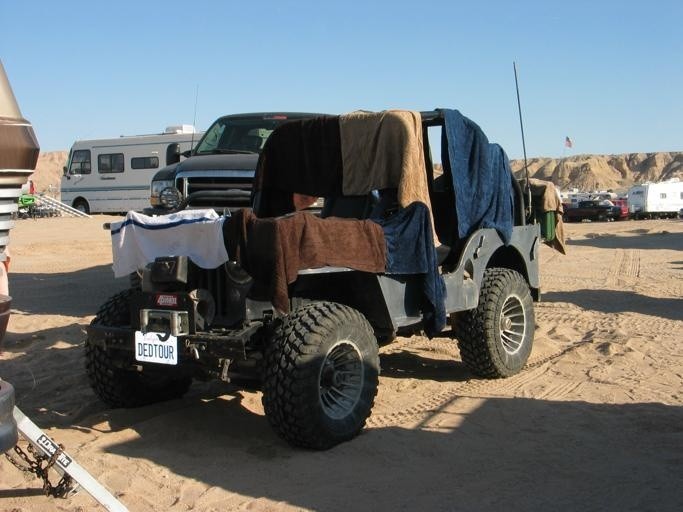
[[566, 136, 573, 148]]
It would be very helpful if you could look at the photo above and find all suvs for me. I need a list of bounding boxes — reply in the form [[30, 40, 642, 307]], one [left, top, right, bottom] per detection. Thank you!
[[613, 199, 627, 220], [153, 112, 337, 211], [84, 109, 544, 455]]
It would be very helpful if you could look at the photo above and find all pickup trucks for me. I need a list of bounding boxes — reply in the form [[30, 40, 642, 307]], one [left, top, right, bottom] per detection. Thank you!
[[563, 200, 622, 222]]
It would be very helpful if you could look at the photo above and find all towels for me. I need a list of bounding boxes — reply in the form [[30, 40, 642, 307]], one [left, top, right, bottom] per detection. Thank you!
[[515, 176, 567, 255], [339, 109, 444, 248], [223, 206, 387, 316], [109, 209, 229, 279], [435, 107, 514, 248], [355, 201, 448, 342]]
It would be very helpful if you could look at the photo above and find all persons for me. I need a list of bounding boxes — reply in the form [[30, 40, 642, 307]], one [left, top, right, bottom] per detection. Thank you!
[[30, 180, 35, 195]]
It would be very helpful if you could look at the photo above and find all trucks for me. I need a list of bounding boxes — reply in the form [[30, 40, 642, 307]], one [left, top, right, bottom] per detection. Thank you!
[[629, 178, 682, 221], [60, 123, 209, 215]]
